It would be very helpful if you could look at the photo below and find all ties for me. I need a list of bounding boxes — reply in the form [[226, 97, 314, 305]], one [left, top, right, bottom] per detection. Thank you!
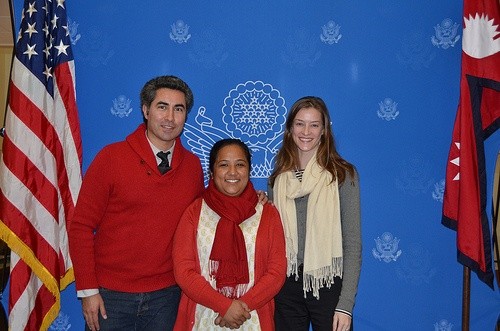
[[156, 150, 172, 174]]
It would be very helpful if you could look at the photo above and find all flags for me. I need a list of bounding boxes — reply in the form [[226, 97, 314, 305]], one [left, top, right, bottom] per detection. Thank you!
[[442, 0, 500, 291], [0, 0, 85, 330]]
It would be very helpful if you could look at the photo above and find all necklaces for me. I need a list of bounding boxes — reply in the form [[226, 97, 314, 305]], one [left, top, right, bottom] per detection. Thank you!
[[295, 166, 304, 176]]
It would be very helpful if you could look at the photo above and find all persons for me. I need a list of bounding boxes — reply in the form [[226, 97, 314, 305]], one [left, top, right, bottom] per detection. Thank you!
[[68, 74, 277, 331], [171, 139, 286, 331], [266, 96, 361, 331]]
